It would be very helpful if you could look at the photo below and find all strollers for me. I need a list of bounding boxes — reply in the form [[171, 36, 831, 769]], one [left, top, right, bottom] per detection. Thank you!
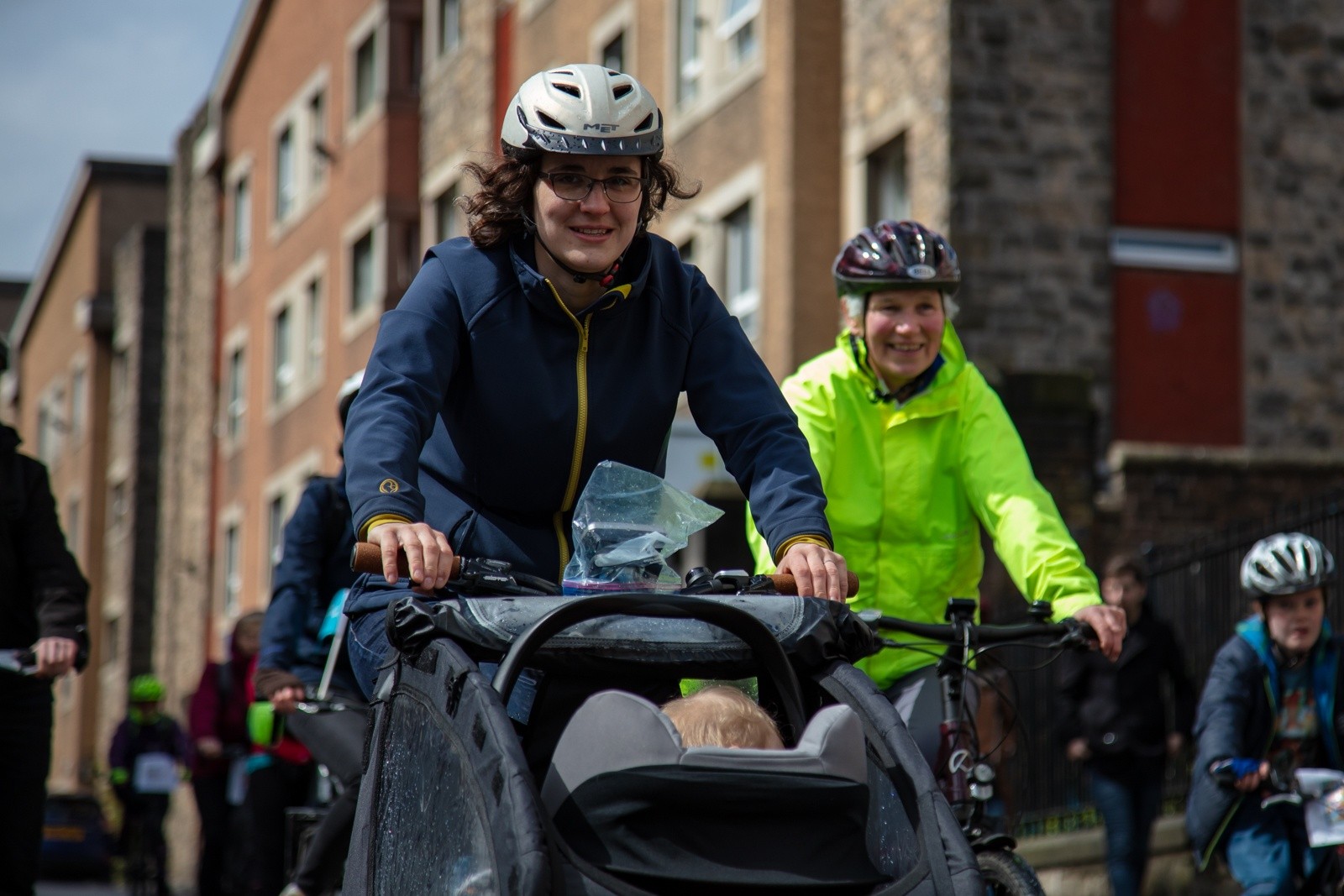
[[338, 592, 987, 896]]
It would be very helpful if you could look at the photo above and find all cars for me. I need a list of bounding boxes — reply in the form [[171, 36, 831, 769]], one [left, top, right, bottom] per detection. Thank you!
[[38, 792, 119, 882]]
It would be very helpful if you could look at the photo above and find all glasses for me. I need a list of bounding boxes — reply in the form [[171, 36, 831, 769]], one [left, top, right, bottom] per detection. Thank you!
[[538, 161, 649, 203]]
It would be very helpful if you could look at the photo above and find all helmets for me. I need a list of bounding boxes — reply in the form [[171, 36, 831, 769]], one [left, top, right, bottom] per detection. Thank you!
[[833, 219, 959, 297], [131, 675, 166, 701], [502, 64, 663, 159], [1240, 534, 1336, 596]]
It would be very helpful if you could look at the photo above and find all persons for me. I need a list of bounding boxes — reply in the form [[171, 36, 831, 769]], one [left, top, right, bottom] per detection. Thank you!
[[661, 684, 783, 750], [254, 370, 369, 896], [345, 62, 848, 896], [0, 337, 92, 896], [747, 221, 1127, 774], [109, 674, 191, 896], [1186, 532, 1344, 896], [1061, 552, 1182, 896], [191, 611, 317, 896]]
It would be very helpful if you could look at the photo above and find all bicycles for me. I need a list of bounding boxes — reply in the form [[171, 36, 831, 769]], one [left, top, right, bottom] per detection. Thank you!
[[1214, 767, 1343, 896], [847, 612, 1098, 896], [337, 542, 985, 598], [124, 697, 375, 891]]
